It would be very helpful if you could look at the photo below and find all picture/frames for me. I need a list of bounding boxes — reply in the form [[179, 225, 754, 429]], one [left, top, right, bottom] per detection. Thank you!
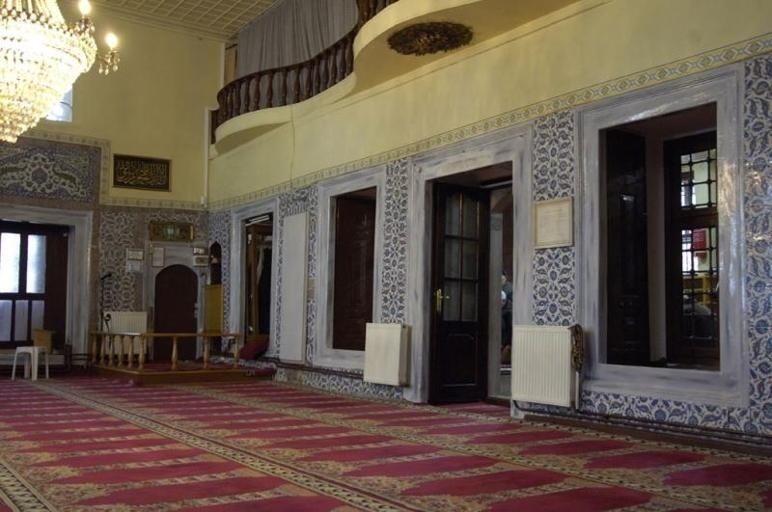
[[529, 197, 576, 251], [148, 220, 195, 242], [113, 151, 175, 193]]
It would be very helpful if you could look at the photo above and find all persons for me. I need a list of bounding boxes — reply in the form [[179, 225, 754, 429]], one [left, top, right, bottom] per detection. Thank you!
[[501, 270, 512, 312]]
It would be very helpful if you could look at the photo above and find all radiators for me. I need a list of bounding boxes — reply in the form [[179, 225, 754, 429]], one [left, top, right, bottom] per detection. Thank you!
[[362, 320, 411, 387], [511, 321, 585, 411], [101, 310, 149, 356]]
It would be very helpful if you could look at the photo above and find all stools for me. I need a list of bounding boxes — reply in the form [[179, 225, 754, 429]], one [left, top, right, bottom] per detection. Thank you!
[[11, 344, 53, 383]]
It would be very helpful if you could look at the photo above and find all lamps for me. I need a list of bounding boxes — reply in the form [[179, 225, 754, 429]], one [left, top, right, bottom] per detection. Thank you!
[[0, 0, 127, 143]]
